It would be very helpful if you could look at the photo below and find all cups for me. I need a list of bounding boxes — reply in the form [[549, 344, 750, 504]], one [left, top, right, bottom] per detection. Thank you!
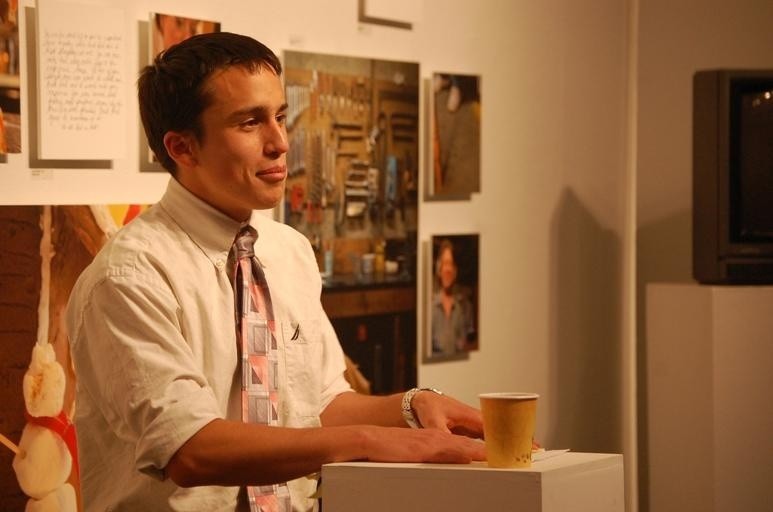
[[477, 391, 539, 469]]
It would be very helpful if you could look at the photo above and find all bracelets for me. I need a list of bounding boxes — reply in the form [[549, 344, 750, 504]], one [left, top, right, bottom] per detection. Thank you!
[[402, 384, 442, 431]]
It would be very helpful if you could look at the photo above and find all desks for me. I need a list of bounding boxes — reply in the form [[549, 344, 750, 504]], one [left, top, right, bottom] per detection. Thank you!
[[321, 274, 416, 393], [321, 452, 625, 511]]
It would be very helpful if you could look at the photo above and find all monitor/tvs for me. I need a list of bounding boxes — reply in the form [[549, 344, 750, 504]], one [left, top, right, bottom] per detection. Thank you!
[[692, 68, 773, 284]]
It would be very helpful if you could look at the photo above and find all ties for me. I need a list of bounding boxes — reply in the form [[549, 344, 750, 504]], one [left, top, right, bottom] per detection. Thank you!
[[230, 224, 294, 511]]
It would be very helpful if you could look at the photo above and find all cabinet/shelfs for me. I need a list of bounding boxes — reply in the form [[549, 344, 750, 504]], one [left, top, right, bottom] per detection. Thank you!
[[641, 282, 773, 512]]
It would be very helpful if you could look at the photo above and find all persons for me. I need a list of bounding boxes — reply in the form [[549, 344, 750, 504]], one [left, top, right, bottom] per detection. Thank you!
[[63, 32, 541, 512], [431, 240, 476, 356], [154, 13, 220, 55]]
